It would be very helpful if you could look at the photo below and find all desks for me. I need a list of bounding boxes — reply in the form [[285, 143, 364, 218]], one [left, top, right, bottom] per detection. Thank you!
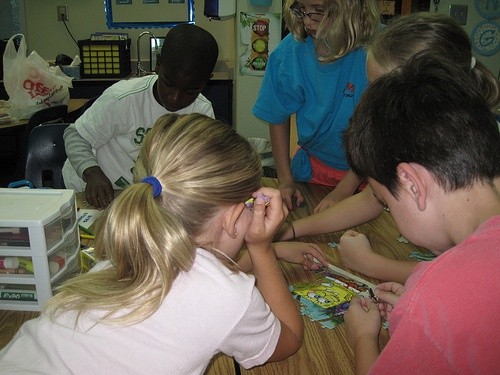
[[0, 119, 29, 180], [0, 177, 429, 375]]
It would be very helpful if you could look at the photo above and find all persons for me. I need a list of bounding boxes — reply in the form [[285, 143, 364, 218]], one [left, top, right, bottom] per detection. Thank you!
[[0, 113, 328, 375], [61, 23, 220, 208], [251, 0, 500, 375]]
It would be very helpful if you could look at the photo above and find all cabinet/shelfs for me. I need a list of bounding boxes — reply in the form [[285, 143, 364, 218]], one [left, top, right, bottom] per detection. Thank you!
[[0, 187, 83, 312]]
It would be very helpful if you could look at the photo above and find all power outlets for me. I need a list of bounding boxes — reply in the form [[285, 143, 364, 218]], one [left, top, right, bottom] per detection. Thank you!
[[56, 3, 68, 21]]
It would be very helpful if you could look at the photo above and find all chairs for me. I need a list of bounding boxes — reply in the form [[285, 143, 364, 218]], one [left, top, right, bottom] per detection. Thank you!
[[23, 94, 99, 187]]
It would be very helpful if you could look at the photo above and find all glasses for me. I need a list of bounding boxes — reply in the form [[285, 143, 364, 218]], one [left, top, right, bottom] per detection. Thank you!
[[289, 1, 325, 23]]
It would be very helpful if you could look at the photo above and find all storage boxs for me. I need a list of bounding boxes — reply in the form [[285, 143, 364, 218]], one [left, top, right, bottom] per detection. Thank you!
[[78, 38, 132, 77]]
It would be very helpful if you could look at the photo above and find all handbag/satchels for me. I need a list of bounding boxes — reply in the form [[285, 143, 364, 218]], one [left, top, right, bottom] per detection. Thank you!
[[4, 34, 74, 120]]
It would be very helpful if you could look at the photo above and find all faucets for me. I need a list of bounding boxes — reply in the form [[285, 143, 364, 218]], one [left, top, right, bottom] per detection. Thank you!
[[136, 30, 158, 76]]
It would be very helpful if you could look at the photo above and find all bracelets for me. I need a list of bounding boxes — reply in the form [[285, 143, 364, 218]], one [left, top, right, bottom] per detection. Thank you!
[[288, 221, 296, 240]]
